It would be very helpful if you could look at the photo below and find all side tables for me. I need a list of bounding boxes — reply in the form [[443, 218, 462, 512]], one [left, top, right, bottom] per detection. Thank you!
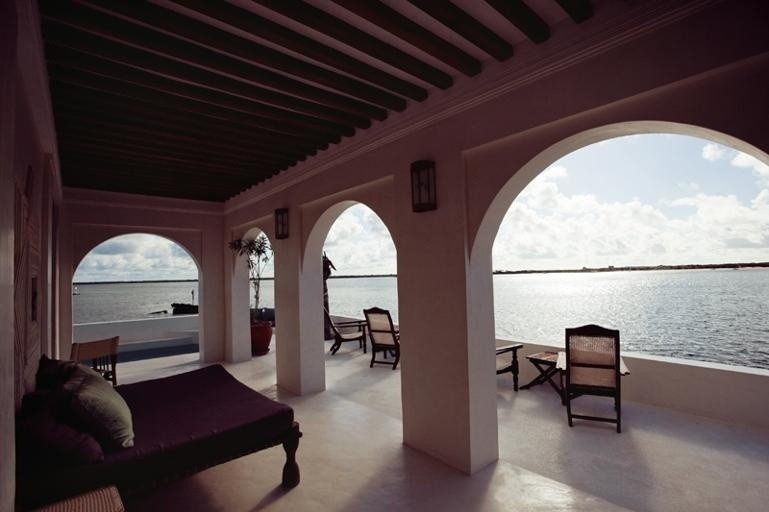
[[520, 351, 567, 406]]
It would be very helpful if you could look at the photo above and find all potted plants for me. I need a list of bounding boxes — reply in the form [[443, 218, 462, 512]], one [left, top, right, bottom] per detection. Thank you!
[[225, 234, 274, 356]]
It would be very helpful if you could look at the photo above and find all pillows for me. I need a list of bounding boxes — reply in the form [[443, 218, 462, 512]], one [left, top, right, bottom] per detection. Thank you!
[[21, 353, 136, 463]]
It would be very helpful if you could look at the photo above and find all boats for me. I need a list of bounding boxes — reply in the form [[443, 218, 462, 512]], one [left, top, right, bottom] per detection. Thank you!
[[72, 286, 80, 295], [171, 288, 199, 315]]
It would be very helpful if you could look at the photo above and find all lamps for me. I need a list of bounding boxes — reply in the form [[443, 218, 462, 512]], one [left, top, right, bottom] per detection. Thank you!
[[411, 161, 437, 212], [275, 208, 288, 239]]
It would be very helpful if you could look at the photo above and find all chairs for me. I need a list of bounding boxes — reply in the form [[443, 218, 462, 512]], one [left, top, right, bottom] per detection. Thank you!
[[324, 307, 368, 355], [496, 344, 523, 391], [555, 325, 630, 433], [363, 307, 400, 370], [71, 337, 119, 384]]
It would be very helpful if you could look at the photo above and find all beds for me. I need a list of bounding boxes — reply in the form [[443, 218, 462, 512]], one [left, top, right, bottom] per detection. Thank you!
[[16, 364, 302, 512]]
